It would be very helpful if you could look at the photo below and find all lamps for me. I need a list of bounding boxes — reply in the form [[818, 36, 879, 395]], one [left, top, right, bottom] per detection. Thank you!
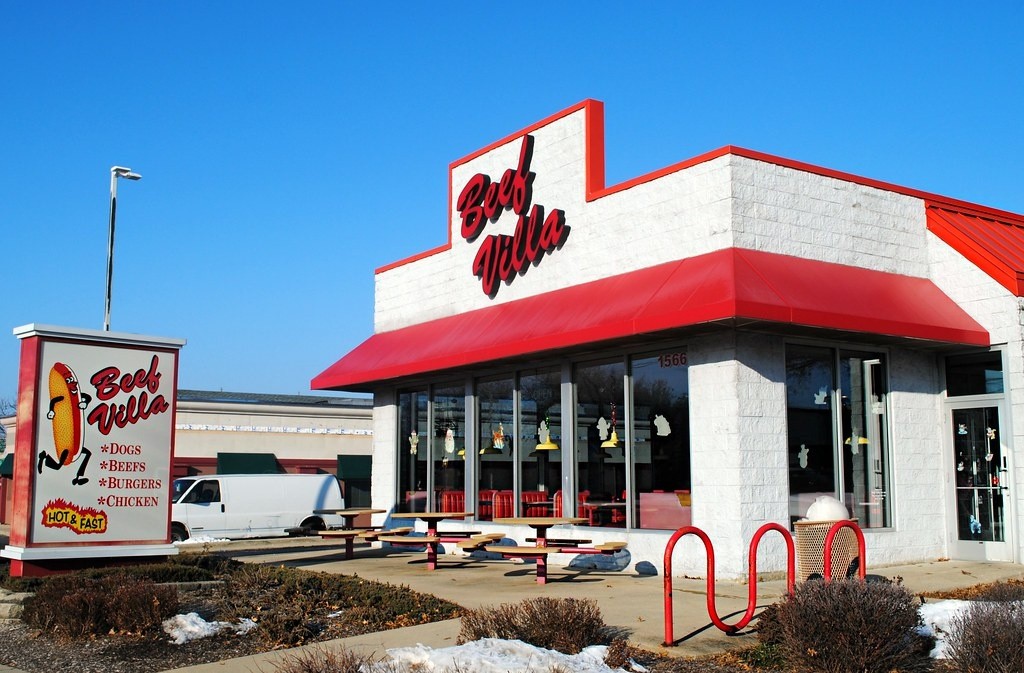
[[535, 374, 560, 450], [457, 449, 465, 459], [601, 367, 624, 448], [479, 384, 503, 455]]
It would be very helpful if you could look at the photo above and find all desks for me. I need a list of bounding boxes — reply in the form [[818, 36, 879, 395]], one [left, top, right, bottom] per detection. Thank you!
[[491, 517, 595, 585], [478, 500, 492, 521], [676, 493, 691, 507], [313, 507, 386, 560], [528, 501, 554, 518], [583, 502, 626, 526], [389, 512, 475, 571]]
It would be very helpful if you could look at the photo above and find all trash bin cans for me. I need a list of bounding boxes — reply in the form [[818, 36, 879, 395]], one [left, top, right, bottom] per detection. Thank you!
[[793, 518, 859, 583]]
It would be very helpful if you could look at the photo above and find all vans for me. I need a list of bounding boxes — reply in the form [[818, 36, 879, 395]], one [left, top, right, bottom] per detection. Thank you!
[[170, 474, 347, 544]]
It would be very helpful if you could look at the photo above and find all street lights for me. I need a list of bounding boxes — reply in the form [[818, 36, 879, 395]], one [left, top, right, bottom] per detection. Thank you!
[[102, 165, 143, 332]]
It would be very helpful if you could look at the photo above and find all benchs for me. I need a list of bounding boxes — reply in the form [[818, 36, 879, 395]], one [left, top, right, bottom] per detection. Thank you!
[[791, 492, 859, 525], [377, 536, 440, 547], [358, 526, 415, 541], [552, 490, 616, 517], [491, 491, 549, 517], [311, 529, 367, 540], [486, 545, 562, 559], [637, 492, 691, 529], [438, 490, 498, 521], [455, 533, 506, 553], [594, 541, 628, 556], [326, 523, 386, 531], [425, 531, 482, 543], [525, 538, 592, 548]]
